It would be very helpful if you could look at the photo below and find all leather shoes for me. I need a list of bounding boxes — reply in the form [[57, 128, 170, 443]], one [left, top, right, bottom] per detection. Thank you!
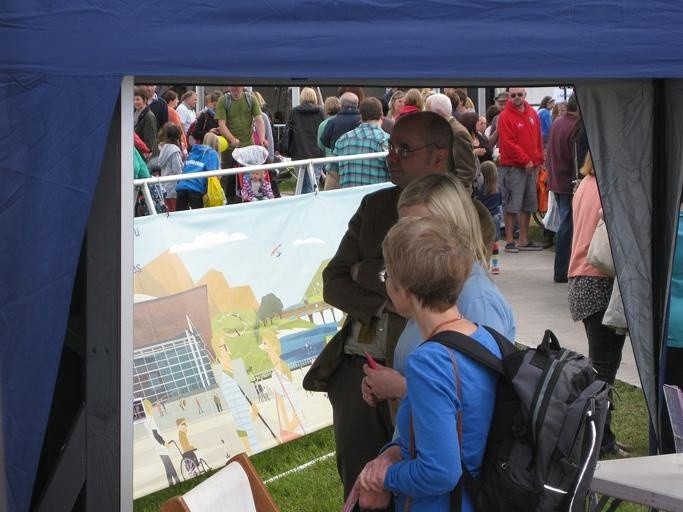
[[613, 448, 630, 458], [617, 443, 632, 451]]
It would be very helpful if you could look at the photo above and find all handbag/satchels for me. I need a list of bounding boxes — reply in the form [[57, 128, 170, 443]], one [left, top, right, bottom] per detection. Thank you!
[[586, 217, 617, 277]]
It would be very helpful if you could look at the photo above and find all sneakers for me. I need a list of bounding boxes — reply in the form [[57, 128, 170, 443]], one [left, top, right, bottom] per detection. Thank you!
[[488, 264, 502, 275], [504, 243, 518, 253], [519, 242, 543, 251]]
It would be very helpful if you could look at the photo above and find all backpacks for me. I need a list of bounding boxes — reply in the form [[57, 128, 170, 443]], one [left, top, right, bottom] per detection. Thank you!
[[425, 325, 612, 511]]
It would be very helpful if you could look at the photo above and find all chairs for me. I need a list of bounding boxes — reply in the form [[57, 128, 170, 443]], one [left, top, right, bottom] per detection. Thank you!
[[662, 384, 683, 453], [157, 452, 280, 512]]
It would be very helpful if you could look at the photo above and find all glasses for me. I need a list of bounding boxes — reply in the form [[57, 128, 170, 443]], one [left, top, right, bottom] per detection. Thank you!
[[388, 142, 440, 156], [379, 268, 403, 285], [510, 92, 524, 98]]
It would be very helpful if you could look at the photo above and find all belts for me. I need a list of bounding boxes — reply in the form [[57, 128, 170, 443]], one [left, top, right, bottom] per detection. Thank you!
[[342, 352, 387, 372]]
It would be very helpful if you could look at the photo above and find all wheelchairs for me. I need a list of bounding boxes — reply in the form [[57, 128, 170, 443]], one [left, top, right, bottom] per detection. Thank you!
[[165, 439, 211, 480]]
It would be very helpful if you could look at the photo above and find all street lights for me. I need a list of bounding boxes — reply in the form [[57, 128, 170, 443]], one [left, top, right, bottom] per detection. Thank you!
[[558, 83, 573, 102]]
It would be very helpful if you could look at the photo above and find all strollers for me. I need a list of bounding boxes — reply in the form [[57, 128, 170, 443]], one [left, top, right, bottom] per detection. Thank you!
[[235, 141, 275, 203]]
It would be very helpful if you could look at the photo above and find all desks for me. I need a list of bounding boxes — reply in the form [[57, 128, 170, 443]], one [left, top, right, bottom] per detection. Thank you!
[[587, 451, 683, 512]]
